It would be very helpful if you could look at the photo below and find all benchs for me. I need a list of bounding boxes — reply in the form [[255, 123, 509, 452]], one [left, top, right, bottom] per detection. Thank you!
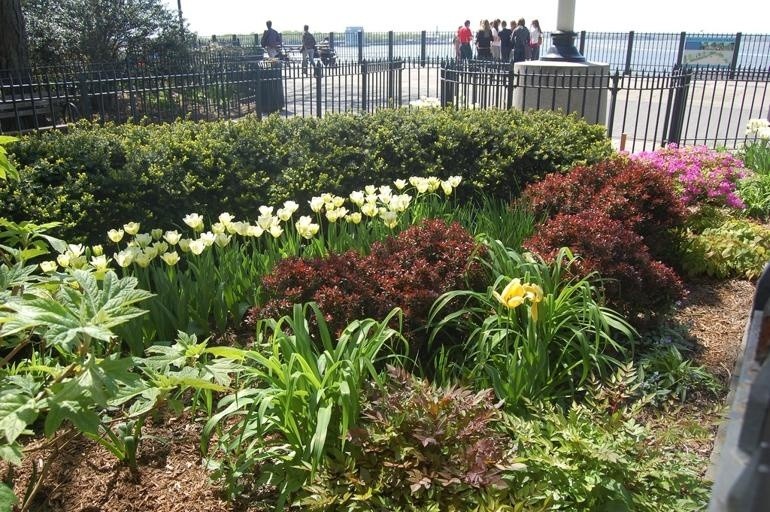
[[280, 44, 337, 63]]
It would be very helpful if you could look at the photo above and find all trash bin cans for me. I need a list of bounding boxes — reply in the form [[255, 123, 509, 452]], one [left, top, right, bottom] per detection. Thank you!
[[256, 64, 284, 110]]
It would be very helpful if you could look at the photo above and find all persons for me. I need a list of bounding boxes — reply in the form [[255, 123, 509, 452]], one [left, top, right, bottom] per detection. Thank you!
[[302, 24, 316, 75], [261, 21, 281, 58], [211, 35, 217, 43], [231, 35, 238, 46], [453, 17, 543, 61], [320, 37, 331, 66]]
